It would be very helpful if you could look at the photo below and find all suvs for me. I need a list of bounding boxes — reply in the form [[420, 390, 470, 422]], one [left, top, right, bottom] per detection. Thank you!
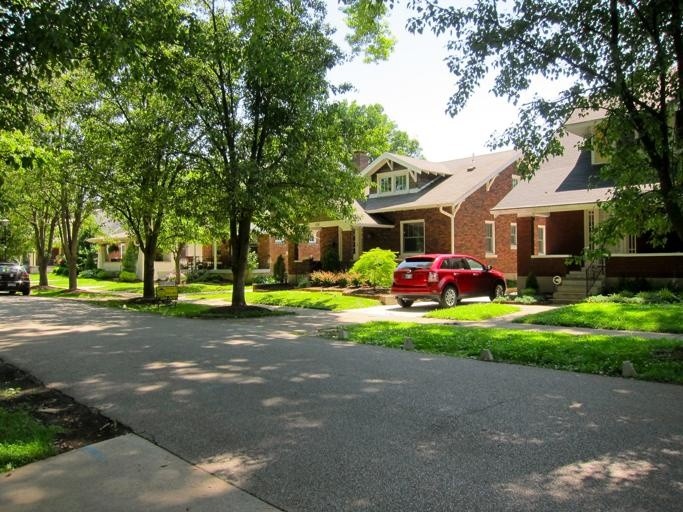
[[390, 255, 507, 307], [0, 263, 30, 296]]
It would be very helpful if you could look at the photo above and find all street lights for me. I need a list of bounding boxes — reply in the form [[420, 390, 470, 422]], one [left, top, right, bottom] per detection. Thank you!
[[0, 219, 10, 261]]
